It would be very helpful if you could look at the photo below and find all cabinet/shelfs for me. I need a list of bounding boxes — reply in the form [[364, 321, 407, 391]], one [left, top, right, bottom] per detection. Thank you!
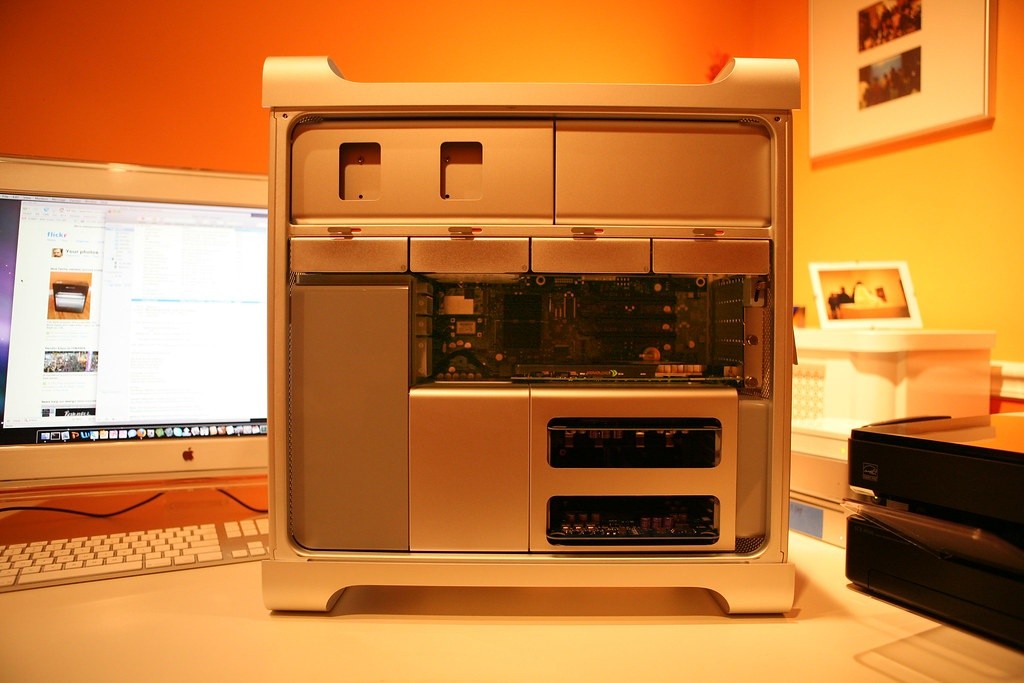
[[790, 327, 996, 546]]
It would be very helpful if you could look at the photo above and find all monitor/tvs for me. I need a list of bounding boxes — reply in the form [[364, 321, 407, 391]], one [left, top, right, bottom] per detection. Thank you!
[[0, 156, 271, 496]]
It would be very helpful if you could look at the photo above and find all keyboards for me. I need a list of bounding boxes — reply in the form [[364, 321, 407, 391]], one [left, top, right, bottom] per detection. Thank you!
[[0, 517, 272, 592]]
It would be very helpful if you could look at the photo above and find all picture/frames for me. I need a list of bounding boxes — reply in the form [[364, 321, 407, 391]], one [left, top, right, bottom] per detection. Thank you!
[[809, 263, 922, 331], [809, 0, 995, 161]]
